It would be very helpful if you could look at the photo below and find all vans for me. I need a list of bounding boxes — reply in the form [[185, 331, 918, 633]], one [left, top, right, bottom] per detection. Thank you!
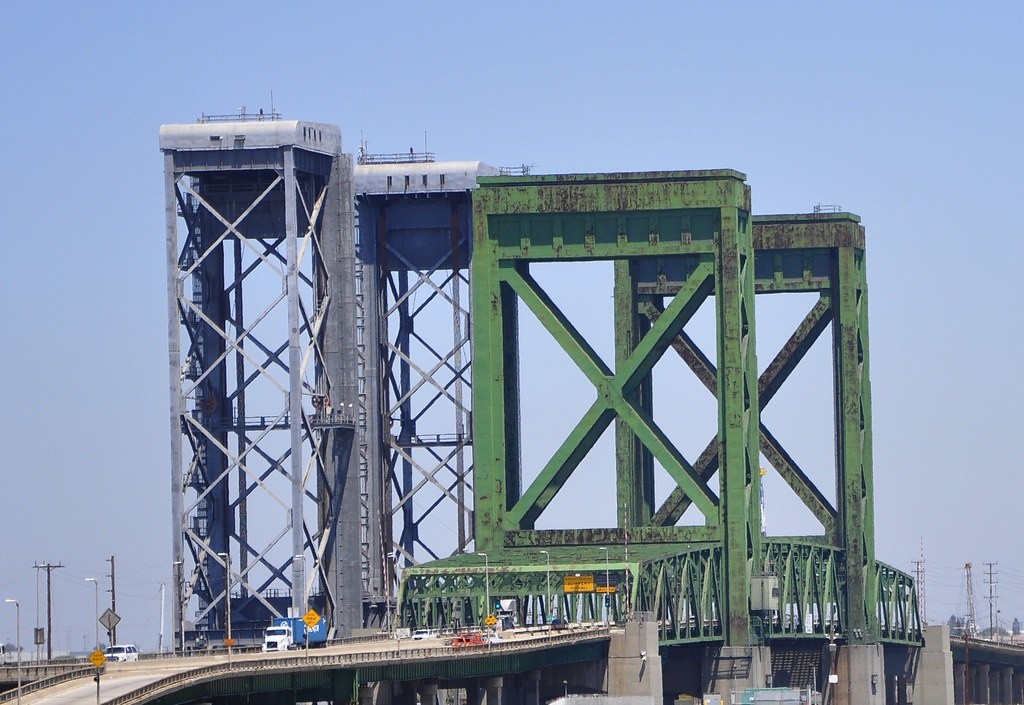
[[411, 628, 442, 640], [101, 646, 139, 662]]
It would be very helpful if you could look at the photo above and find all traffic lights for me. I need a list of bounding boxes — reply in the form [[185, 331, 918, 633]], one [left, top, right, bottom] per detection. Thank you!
[[478, 552, 491, 651], [605, 595, 609, 607], [540, 551, 551, 624], [495, 599, 500, 609], [599, 546, 611, 625]]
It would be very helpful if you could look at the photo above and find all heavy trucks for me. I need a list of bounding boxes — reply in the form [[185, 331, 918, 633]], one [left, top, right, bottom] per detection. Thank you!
[[263, 613, 327, 653]]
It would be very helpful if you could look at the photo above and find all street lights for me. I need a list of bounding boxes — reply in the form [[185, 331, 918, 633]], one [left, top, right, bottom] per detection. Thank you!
[[4, 599, 20, 705], [86, 577, 100, 650], [218, 552, 232, 661]]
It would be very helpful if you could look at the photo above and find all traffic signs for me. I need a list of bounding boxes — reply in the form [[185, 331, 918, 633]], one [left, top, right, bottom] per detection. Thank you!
[[90, 650, 106, 666]]
